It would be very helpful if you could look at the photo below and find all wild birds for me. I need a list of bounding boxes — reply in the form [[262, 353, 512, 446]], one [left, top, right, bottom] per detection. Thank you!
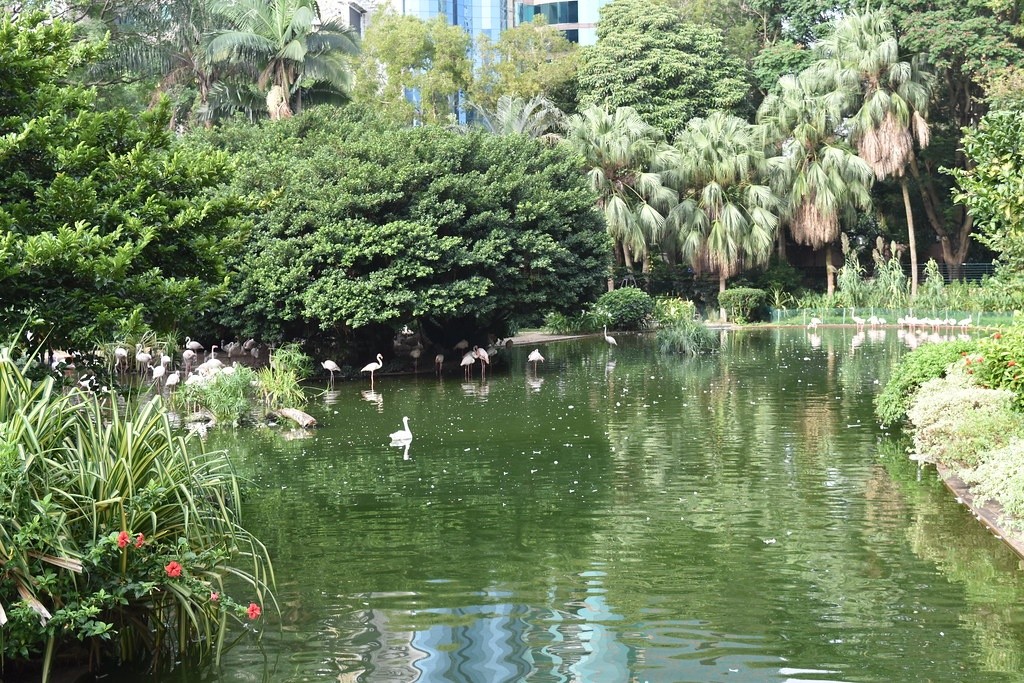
[[321, 360, 341, 381], [454, 332, 514, 383], [849, 306, 973, 327], [360, 353, 384, 386], [50, 333, 289, 416], [409, 341, 444, 378], [389, 416, 413, 441], [527, 348, 545, 372], [807, 312, 822, 330], [602, 324, 617, 348]]
